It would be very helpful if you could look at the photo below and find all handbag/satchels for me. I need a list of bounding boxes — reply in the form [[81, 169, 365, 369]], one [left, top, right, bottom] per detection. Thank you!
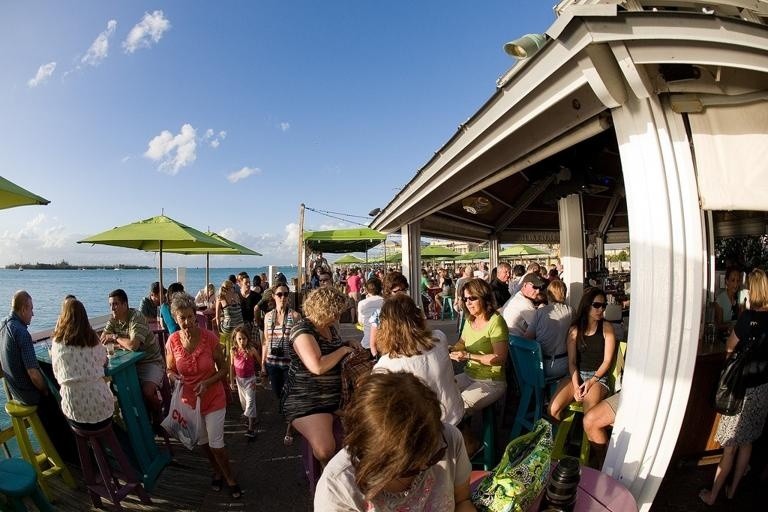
[[341, 347, 375, 409], [469, 418, 552, 512], [711, 310, 759, 415]]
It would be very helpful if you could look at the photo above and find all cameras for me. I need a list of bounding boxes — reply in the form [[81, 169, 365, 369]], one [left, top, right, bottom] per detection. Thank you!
[[271, 347, 284, 357]]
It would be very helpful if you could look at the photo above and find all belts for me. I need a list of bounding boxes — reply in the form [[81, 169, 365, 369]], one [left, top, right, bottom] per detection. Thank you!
[[544, 352, 567, 359]]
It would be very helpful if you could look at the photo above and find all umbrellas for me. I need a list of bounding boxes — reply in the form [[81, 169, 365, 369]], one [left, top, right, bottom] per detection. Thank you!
[[385, 254, 402, 263], [364, 257, 379, 265], [0, 176, 51, 211], [434, 256, 455, 262], [77, 208, 229, 306], [334, 254, 363, 264], [163, 225, 263, 303], [456, 251, 489, 265], [499, 244, 544, 263], [422, 242, 461, 270]]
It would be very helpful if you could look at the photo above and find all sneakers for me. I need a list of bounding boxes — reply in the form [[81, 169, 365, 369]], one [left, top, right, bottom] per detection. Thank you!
[[244, 419, 255, 427], [244, 430, 256, 437]]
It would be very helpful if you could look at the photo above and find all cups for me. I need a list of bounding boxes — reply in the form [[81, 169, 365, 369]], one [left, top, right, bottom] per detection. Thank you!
[[107, 342, 114, 356]]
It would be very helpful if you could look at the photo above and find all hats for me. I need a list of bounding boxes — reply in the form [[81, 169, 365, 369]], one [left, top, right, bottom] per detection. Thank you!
[[150, 282, 167, 295], [223, 280, 236, 288], [523, 273, 545, 287], [275, 271, 288, 284]]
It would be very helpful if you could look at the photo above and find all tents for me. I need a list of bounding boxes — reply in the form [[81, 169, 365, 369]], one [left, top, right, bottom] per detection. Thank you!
[[304, 228, 388, 265]]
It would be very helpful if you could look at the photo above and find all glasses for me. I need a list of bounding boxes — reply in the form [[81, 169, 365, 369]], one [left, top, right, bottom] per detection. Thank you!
[[591, 302, 608, 308], [390, 290, 407, 293], [318, 279, 330, 282], [528, 284, 542, 289], [273, 293, 288, 297], [462, 296, 480, 302], [358, 427, 448, 479]]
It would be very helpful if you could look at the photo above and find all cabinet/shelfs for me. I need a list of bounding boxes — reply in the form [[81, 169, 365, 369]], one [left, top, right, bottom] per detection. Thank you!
[[671, 343, 729, 469]]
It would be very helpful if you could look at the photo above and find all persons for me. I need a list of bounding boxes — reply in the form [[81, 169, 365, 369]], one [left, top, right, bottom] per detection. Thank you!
[[52, 299, 145, 482], [100, 290, 165, 429], [369, 271, 409, 358], [254, 286, 276, 377], [499, 274, 545, 334], [139, 282, 164, 323], [715, 268, 740, 330], [229, 326, 262, 438], [307, 251, 383, 288], [448, 278, 509, 462], [698, 268, 767, 507], [582, 390, 621, 468], [420, 261, 489, 313], [357, 278, 384, 350], [547, 286, 616, 422], [345, 268, 363, 323], [371, 294, 464, 428], [166, 292, 241, 501], [227, 272, 289, 292], [313, 368, 476, 512], [261, 283, 302, 447], [195, 283, 214, 304], [524, 279, 576, 378], [215, 280, 244, 337], [0, 290, 75, 467], [492, 262, 512, 306], [281, 286, 363, 466], [239, 275, 262, 326], [509, 263, 563, 296], [159, 283, 184, 334]]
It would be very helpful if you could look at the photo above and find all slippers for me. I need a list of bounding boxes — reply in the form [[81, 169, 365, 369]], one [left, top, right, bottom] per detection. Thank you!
[[469, 443, 485, 460], [724, 481, 734, 501], [210, 472, 222, 492], [227, 478, 242, 500], [696, 484, 714, 509]]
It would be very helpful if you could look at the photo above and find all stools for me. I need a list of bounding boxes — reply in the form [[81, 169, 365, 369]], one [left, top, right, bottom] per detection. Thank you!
[[71, 422, 152, 512], [302, 415, 345, 495], [470, 409, 493, 471], [0, 457, 50, 512], [5, 400, 76, 504], [441, 295, 455, 321]]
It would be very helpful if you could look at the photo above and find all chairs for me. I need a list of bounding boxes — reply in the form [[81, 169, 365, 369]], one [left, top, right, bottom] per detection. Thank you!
[[506, 335, 563, 443], [549, 340, 628, 465]]
